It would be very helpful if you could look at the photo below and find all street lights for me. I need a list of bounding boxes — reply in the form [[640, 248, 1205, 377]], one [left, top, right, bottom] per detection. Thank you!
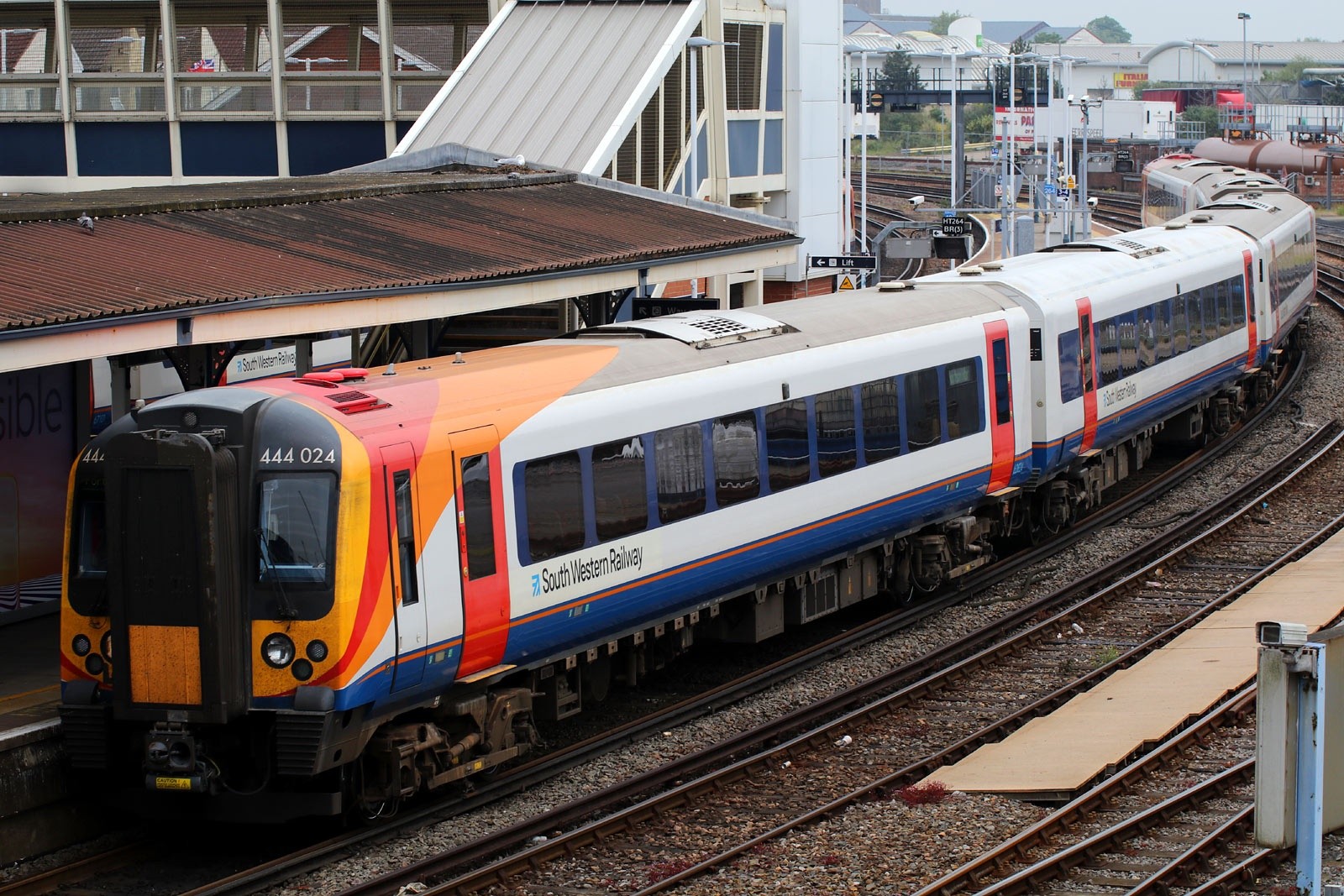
[[686, 37, 740, 201], [1255, 41, 1273, 81], [1179, 42, 1219, 82], [1058, 38, 1083, 99], [1067, 95, 1103, 241], [1237, 13, 1252, 140], [921, 49, 979, 273], [844, 44, 912, 270], [984, 52, 1087, 258], [1112, 53, 1120, 100]]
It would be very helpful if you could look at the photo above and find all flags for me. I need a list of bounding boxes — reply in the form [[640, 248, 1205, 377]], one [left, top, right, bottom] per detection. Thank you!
[[188, 59, 214, 73]]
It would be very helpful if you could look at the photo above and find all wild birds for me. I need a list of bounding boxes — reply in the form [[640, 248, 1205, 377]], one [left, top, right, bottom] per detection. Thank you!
[[78, 213, 94, 233]]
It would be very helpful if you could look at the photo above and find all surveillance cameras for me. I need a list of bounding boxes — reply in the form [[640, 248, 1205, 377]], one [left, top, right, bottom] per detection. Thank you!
[[1097, 97, 1102, 102], [1067, 94, 1074, 103], [1086, 197, 1098, 207], [907, 195, 925, 205], [1081, 95, 1090, 102]]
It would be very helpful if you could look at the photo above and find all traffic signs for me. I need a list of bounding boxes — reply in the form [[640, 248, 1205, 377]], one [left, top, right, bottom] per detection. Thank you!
[[809, 255, 878, 271]]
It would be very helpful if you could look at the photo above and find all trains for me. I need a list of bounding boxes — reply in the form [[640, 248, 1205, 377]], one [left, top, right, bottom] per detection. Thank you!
[[57, 149, 1318, 870]]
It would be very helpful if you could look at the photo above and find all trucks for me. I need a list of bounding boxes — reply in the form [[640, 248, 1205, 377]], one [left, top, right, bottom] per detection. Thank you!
[[1142, 87, 1254, 142]]
